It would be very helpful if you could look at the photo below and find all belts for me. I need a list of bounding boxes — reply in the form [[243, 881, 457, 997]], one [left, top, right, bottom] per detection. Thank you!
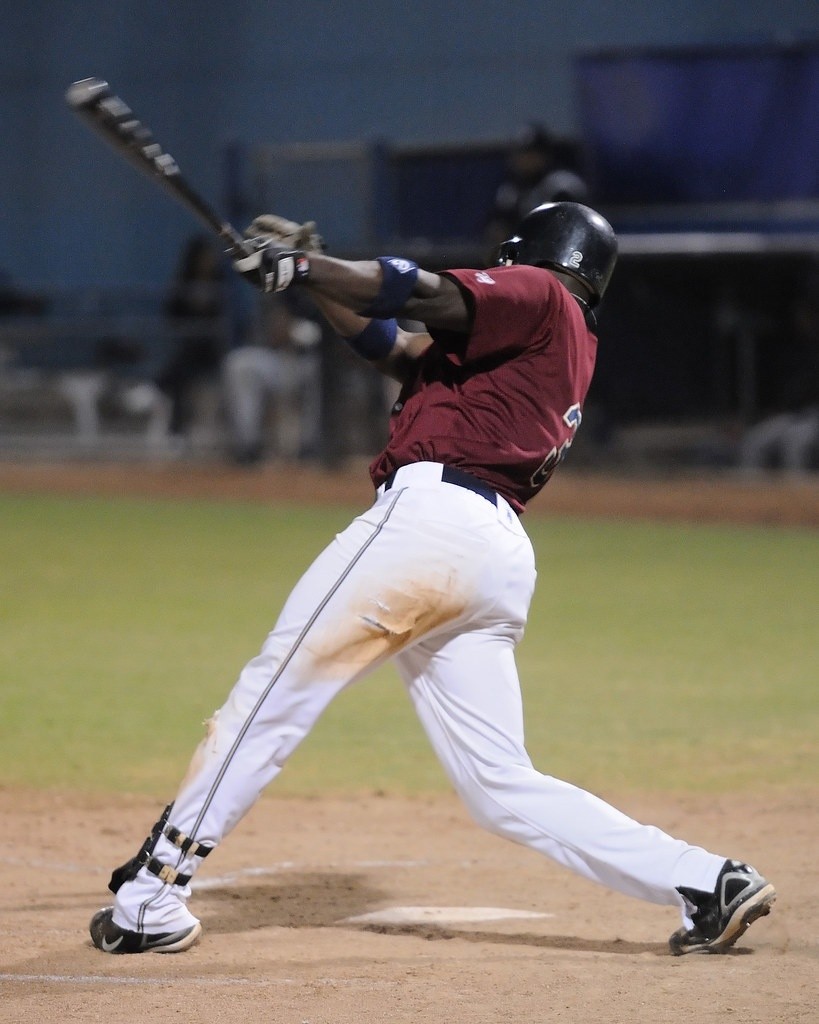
[[384, 466, 497, 507]]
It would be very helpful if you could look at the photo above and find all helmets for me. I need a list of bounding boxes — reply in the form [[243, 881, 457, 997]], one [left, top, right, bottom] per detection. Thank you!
[[497, 201, 619, 314]]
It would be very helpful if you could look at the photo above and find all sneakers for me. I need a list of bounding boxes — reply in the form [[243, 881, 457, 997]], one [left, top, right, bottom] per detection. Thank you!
[[669, 857, 776, 955], [89, 906, 201, 953]]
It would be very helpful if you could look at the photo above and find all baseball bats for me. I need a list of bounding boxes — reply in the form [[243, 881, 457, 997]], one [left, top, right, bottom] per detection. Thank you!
[[68, 75, 256, 261]]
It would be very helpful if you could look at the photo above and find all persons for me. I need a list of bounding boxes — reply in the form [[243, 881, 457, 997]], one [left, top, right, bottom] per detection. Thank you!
[[89, 201, 778, 953]]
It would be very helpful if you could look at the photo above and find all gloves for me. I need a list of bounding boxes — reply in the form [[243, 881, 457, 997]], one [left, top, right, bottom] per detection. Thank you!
[[243, 215, 323, 259], [226, 234, 310, 293]]
